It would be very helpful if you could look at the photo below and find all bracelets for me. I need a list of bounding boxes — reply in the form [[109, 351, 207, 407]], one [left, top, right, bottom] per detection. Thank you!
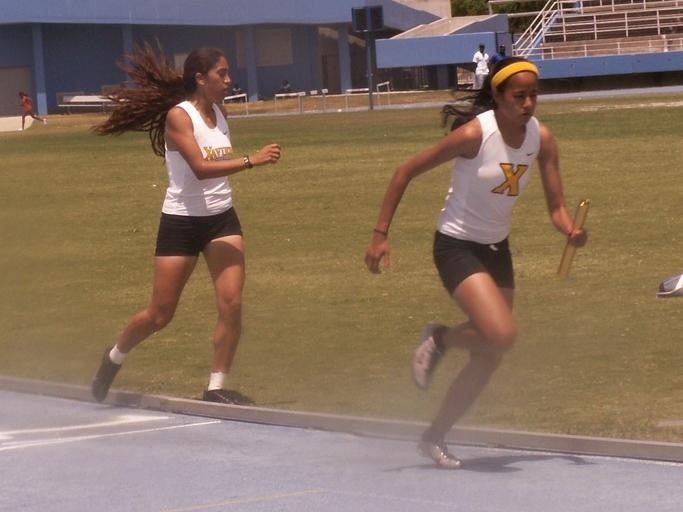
[[372, 227, 389, 238], [242, 155, 255, 167]]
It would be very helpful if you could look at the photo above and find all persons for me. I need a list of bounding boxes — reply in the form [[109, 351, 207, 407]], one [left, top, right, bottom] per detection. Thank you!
[[92, 40, 283, 407], [489, 44, 509, 71], [17, 91, 46, 131], [472, 42, 489, 92], [362, 58, 588, 468]]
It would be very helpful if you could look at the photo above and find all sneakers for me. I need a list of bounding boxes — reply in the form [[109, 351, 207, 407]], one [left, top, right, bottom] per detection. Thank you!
[[417, 437, 462, 469], [412, 322, 447, 389], [92, 348, 120, 401], [203, 388, 252, 407]]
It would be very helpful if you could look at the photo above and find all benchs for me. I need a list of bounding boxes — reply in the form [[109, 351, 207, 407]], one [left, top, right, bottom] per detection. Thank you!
[[59, 95, 131, 112]]
